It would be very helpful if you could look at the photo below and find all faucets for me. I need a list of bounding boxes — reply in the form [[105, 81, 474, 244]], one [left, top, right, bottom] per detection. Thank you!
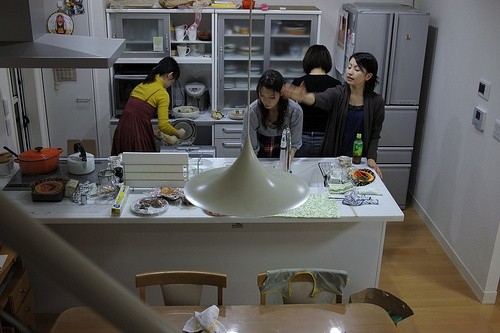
[[280, 127, 292, 173]]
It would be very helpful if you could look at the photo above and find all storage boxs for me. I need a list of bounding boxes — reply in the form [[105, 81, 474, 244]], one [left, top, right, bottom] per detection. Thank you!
[[65, 179, 79, 197], [112, 185, 128, 216]]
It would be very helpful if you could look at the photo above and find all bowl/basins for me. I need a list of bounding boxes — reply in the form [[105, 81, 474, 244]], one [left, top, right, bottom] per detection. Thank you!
[[224, 46, 260, 56], [173, 105, 199, 119], [197, 32, 211, 41], [240, 28, 249, 35], [282, 26, 307, 35], [228, 111, 243, 119], [289, 43, 309, 57], [242, 1, 255, 9]]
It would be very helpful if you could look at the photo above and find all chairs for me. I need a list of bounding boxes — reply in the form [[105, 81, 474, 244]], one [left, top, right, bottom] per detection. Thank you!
[[135, 271, 227, 306], [257, 268, 348, 305]]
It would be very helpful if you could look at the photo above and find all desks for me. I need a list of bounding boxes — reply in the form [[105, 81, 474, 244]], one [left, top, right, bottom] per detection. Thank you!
[[50, 303, 402, 333]]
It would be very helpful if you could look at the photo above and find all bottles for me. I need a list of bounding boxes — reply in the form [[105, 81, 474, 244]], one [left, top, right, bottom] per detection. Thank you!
[[353, 133, 363, 165]]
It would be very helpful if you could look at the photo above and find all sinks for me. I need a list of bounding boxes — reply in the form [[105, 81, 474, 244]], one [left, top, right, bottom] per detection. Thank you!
[[224, 161, 325, 188]]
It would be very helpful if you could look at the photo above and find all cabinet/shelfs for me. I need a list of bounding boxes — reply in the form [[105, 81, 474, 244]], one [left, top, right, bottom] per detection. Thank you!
[[28, 0, 320, 159]]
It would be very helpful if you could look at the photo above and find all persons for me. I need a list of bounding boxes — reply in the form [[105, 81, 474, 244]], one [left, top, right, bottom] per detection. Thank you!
[[282, 52, 384, 178], [240, 69, 302, 159], [289, 45, 342, 157], [112, 56, 187, 156]]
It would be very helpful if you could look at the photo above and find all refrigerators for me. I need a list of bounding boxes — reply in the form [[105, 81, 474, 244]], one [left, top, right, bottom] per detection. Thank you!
[[336, 2, 431, 210]]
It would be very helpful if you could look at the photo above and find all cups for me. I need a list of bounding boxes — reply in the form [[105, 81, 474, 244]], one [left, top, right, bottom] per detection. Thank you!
[[340, 156, 352, 166], [98, 169, 116, 186], [177, 45, 190, 57], [188, 27, 197, 41], [175, 24, 188, 41], [0, 156, 14, 176]]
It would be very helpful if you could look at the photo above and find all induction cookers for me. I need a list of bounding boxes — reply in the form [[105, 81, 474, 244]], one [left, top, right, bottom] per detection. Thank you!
[[2, 159, 110, 191]]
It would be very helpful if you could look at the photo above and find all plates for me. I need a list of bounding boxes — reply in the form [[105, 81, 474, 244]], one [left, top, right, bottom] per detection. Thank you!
[[354, 169, 375, 185], [130, 197, 169, 215], [154, 187, 184, 201]]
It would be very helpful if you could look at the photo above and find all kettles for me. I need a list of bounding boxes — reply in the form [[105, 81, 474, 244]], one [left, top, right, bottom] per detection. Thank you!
[[67, 143, 96, 175]]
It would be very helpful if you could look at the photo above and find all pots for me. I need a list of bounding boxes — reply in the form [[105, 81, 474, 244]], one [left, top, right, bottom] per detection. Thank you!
[[13, 146, 64, 175]]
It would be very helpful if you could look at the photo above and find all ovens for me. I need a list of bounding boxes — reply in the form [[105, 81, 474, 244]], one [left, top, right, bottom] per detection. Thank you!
[[113, 64, 172, 120]]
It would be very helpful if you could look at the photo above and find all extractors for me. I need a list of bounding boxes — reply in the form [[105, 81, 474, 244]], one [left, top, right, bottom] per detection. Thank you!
[[0, 0, 127, 69]]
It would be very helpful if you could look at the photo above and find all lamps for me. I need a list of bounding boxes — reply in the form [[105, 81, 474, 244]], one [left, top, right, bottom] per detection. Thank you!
[[183, 0, 310, 217]]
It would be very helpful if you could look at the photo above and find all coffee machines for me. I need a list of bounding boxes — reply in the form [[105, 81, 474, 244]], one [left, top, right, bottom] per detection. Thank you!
[[184, 81, 207, 114]]
